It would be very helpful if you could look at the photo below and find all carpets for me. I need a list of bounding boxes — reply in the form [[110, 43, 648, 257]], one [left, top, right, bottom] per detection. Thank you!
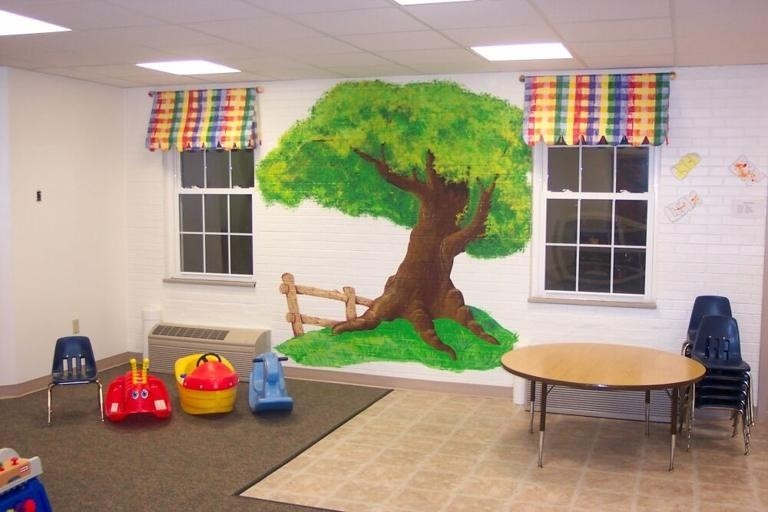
[[1, 360, 393, 512]]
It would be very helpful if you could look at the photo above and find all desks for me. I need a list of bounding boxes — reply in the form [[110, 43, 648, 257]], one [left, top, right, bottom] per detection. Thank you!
[[501, 344, 707, 472]]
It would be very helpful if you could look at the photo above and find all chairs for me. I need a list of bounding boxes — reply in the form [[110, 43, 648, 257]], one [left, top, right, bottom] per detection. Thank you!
[[46, 336, 104, 424], [679, 295, 755, 454]]
